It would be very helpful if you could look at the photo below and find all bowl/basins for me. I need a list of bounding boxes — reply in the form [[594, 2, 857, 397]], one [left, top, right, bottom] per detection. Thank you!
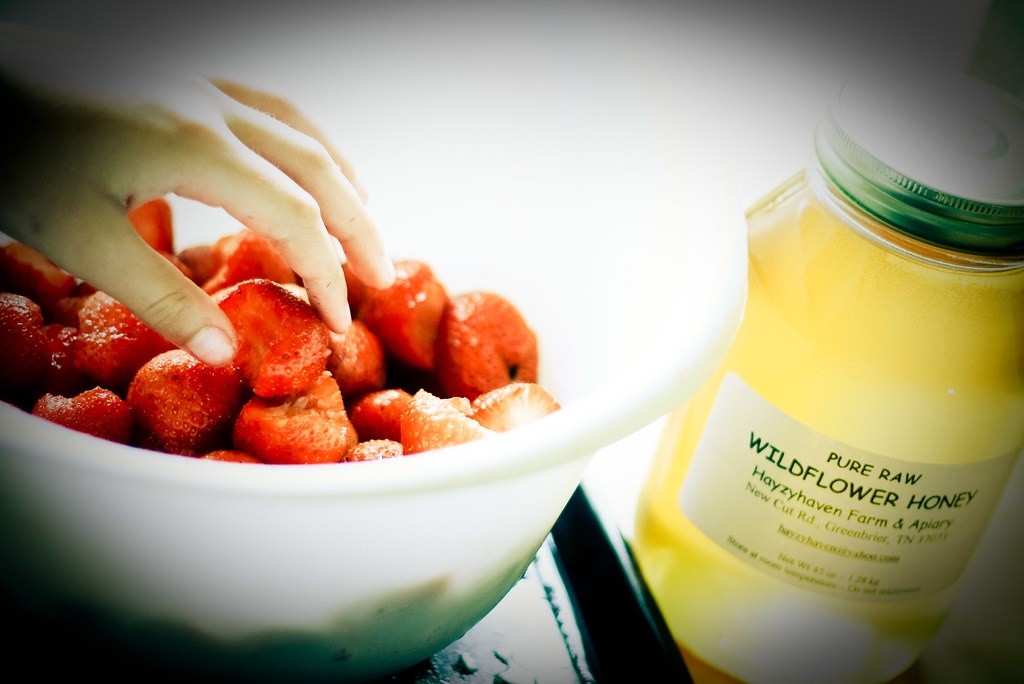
[[0, 179, 750, 677]]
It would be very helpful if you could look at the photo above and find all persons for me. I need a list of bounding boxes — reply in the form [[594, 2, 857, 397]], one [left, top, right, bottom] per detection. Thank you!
[[0, 40, 395, 368]]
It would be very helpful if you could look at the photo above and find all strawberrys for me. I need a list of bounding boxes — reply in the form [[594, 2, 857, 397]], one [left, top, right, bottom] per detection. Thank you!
[[0, 197, 560, 464]]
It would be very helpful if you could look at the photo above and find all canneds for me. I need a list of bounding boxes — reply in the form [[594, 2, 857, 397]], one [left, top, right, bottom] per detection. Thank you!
[[626, 67, 1024, 684]]
